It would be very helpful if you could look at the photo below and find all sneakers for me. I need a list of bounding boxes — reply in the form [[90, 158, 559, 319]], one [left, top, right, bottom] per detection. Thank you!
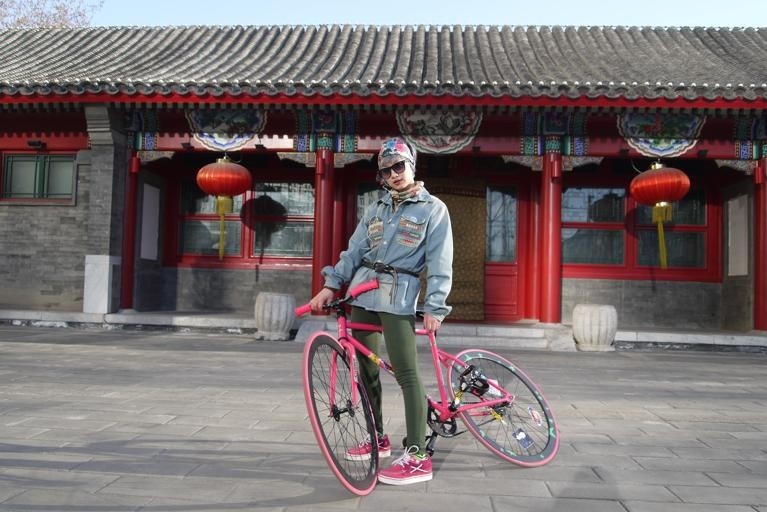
[[377, 445, 433, 485], [344, 434, 392, 461]]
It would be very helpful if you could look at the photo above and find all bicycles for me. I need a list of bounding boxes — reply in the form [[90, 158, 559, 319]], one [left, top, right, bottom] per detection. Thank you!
[[293, 278, 562, 498]]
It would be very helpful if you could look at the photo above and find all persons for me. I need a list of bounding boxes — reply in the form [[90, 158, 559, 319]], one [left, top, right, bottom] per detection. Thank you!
[[310, 136, 454, 485]]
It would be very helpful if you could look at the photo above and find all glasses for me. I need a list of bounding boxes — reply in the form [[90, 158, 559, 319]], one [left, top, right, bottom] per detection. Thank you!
[[379, 159, 408, 179]]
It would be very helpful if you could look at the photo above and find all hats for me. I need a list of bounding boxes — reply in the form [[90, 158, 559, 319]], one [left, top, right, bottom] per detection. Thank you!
[[377, 139, 417, 171]]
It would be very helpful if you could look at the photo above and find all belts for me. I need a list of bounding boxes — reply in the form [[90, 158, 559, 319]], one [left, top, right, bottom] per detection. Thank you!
[[361, 261, 419, 278]]
